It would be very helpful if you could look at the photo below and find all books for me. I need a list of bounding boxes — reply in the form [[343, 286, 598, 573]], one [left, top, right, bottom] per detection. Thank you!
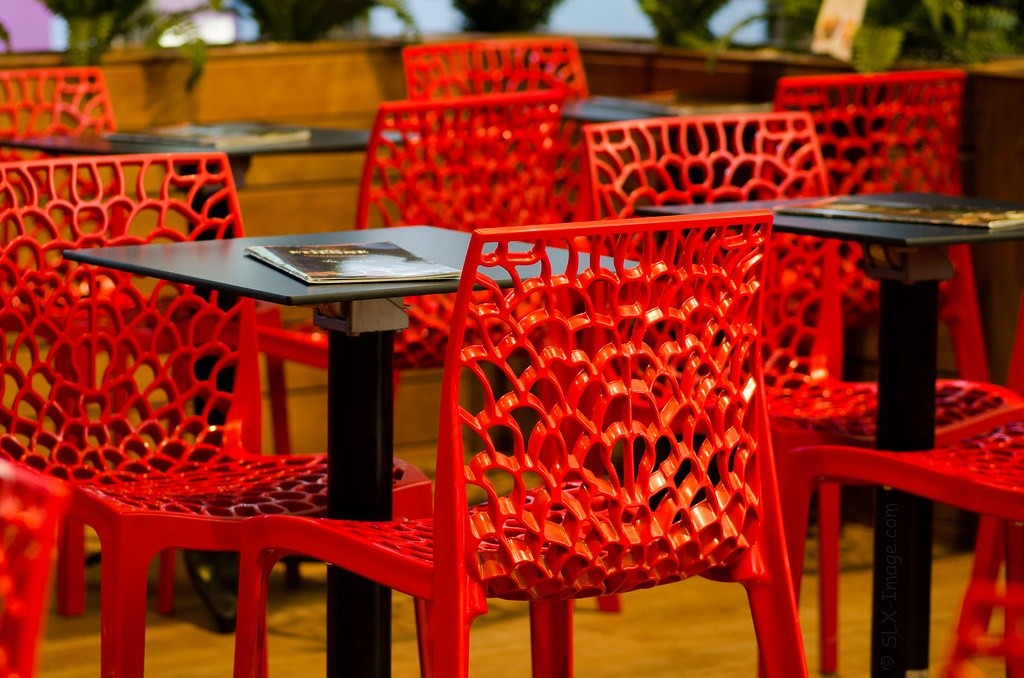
[[774, 192, 1024, 230], [243, 241, 462, 284], [100, 120, 311, 149], [591, 87, 773, 116]]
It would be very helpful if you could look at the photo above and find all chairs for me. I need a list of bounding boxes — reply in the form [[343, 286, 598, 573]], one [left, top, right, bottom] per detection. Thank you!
[[2, 34, 1024, 678]]
